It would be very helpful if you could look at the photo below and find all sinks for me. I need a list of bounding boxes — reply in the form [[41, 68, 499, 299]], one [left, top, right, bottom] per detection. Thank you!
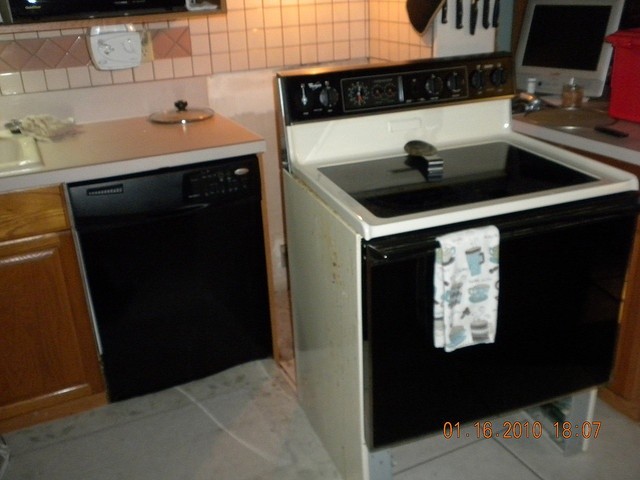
[[0, 129, 59, 177]]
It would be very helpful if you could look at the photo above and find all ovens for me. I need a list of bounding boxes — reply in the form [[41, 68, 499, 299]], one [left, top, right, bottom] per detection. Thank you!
[[272, 52, 639, 479], [66, 153, 274, 404]]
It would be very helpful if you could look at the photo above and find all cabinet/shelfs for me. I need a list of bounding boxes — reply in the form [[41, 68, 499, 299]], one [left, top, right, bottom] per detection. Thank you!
[[0, 186, 106, 442]]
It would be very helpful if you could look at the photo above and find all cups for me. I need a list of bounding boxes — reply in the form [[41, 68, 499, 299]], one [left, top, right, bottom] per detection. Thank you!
[[562, 83, 583, 112]]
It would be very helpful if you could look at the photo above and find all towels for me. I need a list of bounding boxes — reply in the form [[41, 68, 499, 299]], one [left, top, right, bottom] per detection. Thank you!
[[433, 226, 499, 352]]
